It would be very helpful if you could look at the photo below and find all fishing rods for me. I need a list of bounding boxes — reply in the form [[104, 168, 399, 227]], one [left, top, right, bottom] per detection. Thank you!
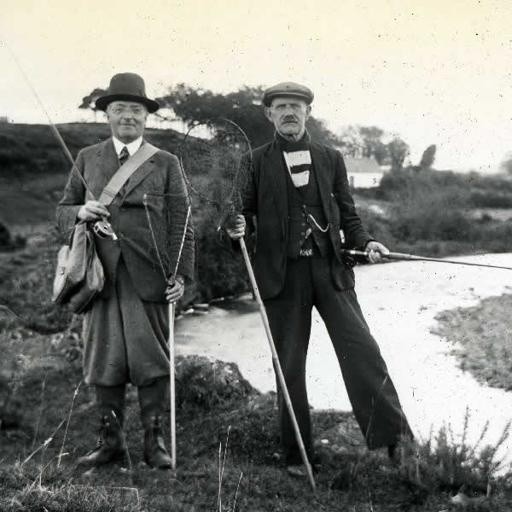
[[0, 32, 117, 241], [339, 247, 512, 270]]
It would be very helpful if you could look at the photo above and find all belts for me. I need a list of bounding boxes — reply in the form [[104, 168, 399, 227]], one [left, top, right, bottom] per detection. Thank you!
[[299, 248, 320, 259]]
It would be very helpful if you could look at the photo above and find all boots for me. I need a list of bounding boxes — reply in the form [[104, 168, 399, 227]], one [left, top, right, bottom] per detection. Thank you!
[[138, 402, 172, 469], [77, 401, 129, 466]]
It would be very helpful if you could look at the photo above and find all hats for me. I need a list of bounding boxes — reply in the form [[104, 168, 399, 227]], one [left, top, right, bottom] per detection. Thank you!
[[95, 73, 159, 113], [260, 83, 314, 107]]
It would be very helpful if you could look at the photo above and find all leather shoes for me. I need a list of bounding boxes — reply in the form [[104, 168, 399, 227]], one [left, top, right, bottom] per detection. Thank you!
[[285, 463, 310, 479]]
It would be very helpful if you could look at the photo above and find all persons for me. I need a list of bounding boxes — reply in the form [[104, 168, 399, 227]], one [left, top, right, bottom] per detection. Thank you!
[[219, 82, 420, 480], [57, 73, 195, 467]]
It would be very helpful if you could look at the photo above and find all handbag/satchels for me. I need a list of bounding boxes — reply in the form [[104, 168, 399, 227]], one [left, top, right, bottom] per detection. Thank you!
[[51, 223, 105, 314]]
